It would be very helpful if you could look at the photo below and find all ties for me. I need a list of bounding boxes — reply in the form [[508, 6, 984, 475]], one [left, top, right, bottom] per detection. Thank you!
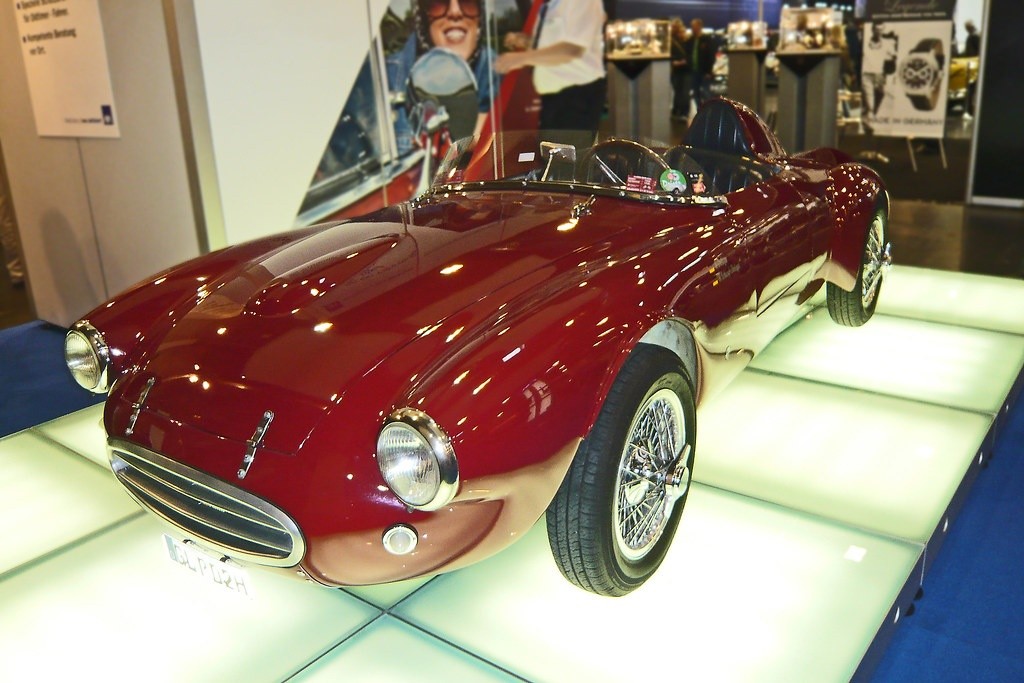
[[530, 1, 548, 50]]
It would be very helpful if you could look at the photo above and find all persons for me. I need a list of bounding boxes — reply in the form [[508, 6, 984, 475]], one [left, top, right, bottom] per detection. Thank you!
[[863, 20, 897, 118], [494, 1, 609, 162], [685, 19, 719, 111], [963, 22, 980, 116], [671, 19, 693, 115], [384, 0, 502, 156]]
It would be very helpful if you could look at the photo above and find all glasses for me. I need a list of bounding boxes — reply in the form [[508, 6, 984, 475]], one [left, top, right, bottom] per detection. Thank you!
[[422, 0, 482, 20]]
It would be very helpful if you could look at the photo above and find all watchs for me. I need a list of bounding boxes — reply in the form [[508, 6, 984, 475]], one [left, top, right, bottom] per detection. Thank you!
[[899, 38, 945, 110]]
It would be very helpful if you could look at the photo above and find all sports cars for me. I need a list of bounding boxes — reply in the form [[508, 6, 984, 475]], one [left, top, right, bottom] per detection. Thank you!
[[63, 94, 894, 602]]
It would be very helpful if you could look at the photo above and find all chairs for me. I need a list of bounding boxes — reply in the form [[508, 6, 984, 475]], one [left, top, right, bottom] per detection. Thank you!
[[572, 146, 671, 192]]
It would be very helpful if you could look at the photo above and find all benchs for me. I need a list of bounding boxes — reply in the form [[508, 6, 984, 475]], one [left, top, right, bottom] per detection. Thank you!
[[654, 95, 782, 199]]
